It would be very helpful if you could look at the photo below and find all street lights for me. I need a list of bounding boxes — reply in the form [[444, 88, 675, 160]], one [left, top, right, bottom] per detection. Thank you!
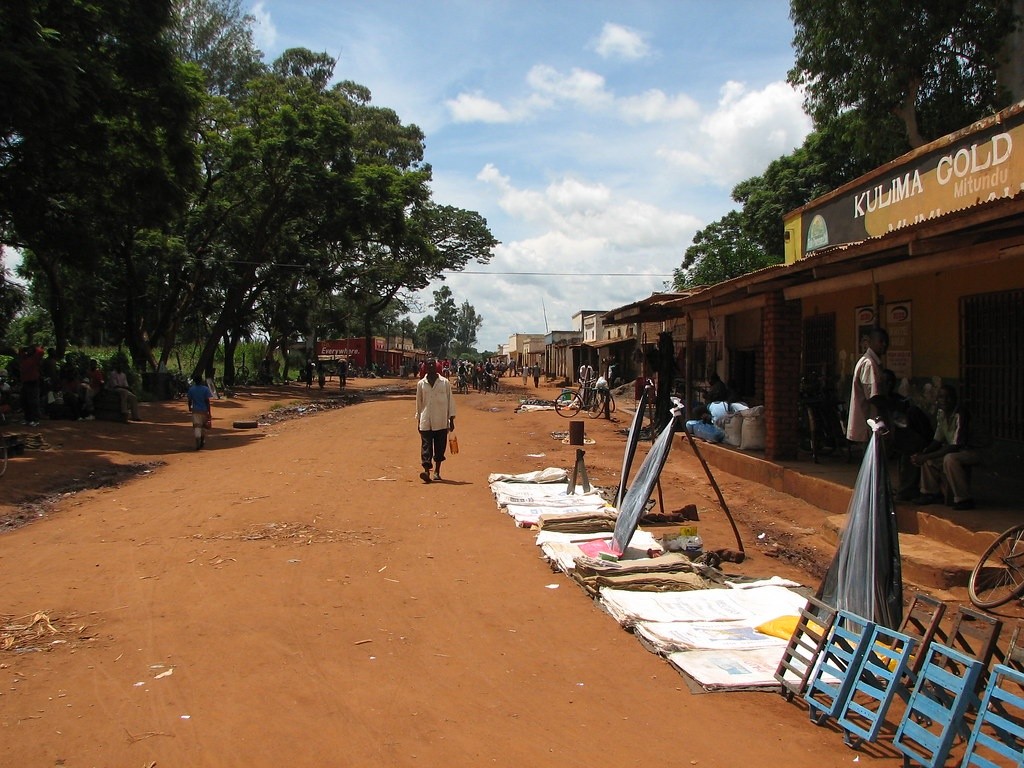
[[385, 315, 392, 376]]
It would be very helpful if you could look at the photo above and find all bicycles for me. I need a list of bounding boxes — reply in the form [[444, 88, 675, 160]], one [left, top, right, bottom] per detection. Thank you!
[[967, 521, 1024, 608], [484, 377, 502, 396], [0, 376, 15, 478], [457, 373, 470, 395], [554, 378, 616, 418]]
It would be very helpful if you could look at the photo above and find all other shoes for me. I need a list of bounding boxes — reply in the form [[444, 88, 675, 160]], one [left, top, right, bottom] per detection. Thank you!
[[77, 416, 86, 421], [119, 413, 129, 419], [946, 498, 975, 510], [85, 414, 96, 421], [18, 419, 30, 425], [131, 418, 142, 421], [433, 472, 441, 480], [29, 421, 40, 427], [911, 493, 945, 505], [197, 446, 201, 450], [419, 473, 432, 483], [201, 439, 205, 445], [894, 488, 921, 502]]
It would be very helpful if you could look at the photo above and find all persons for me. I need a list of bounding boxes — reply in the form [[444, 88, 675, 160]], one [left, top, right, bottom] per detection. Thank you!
[[847, 328, 983, 511], [337, 360, 346, 392], [0, 369, 10, 390], [306, 361, 314, 390], [578, 360, 594, 386], [317, 367, 325, 389], [692, 370, 727, 420], [187, 374, 211, 448], [258, 363, 274, 386], [415, 358, 455, 481], [20, 346, 143, 428], [347, 360, 395, 378], [442, 355, 541, 394]]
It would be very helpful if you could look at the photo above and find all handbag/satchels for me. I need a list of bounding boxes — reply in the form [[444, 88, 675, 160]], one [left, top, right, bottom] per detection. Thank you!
[[203, 409, 211, 430]]
